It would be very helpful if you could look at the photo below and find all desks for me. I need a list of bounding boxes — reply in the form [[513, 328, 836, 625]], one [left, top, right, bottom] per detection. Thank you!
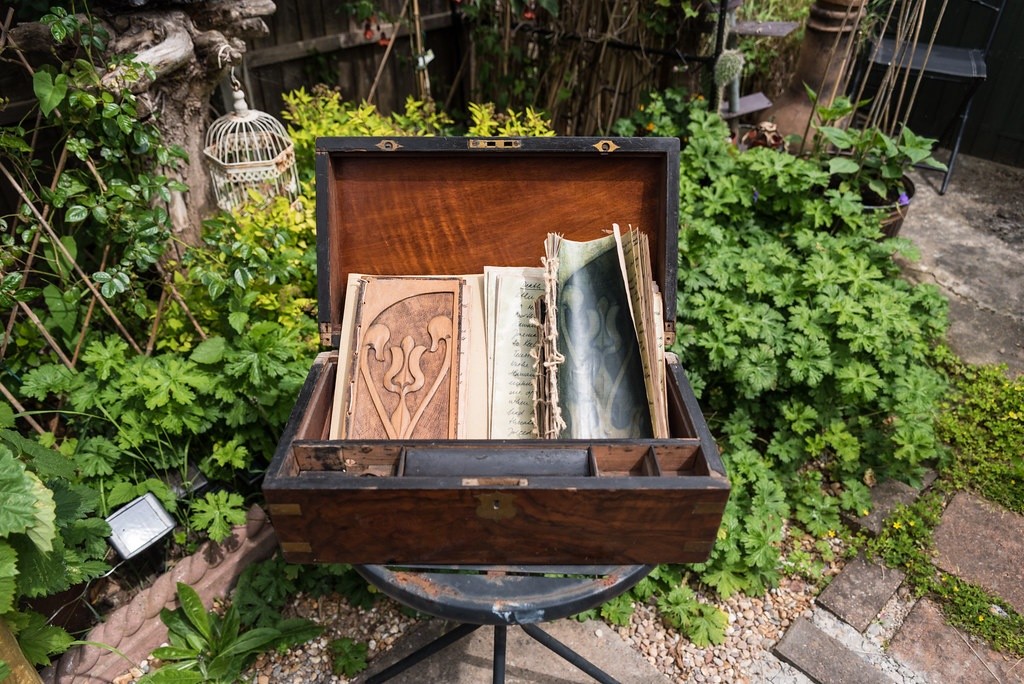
[[345, 564, 663, 684]]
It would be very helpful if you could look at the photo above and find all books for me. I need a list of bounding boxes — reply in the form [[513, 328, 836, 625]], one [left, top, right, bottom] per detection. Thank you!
[[329, 223, 671, 439]]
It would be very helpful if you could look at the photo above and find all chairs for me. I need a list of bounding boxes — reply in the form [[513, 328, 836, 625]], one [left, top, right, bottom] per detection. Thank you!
[[839, 0, 1008, 195]]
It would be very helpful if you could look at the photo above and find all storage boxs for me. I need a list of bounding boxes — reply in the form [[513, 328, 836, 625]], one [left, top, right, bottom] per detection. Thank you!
[[260, 136, 731, 564]]
